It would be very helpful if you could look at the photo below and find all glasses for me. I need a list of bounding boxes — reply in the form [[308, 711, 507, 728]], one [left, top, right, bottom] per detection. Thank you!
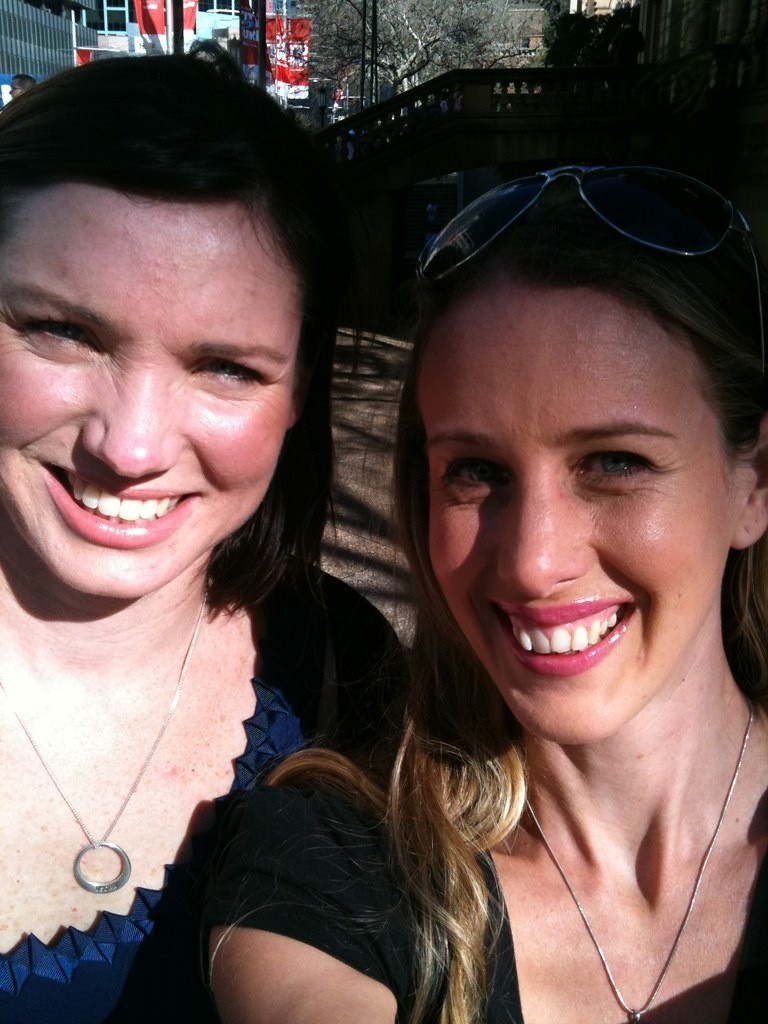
[[417, 164, 768, 373]]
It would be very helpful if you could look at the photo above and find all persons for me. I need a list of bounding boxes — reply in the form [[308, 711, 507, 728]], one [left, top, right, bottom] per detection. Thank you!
[[9, 73, 36, 97], [1, 37, 414, 1024], [210, 162, 767, 1024]]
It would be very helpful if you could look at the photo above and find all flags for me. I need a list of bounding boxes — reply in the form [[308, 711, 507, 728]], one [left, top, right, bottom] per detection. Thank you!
[[182, 0, 197, 30], [133, 0, 166, 35], [76, 47, 91, 67], [266, 11, 349, 113], [239, 0, 260, 80]]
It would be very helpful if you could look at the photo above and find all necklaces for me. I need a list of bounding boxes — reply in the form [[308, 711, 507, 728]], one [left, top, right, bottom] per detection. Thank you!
[[524, 692, 753, 1021], [3, 590, 209, 895]]
